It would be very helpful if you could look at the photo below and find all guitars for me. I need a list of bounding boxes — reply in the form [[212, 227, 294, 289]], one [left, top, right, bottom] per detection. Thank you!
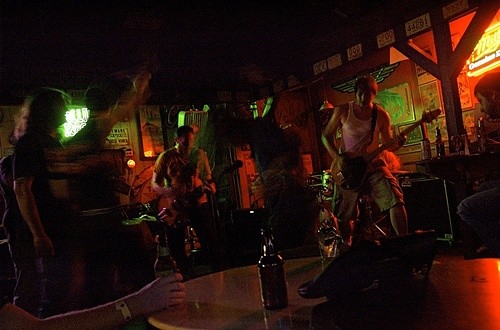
[[157, 160, 243, 225], [330, 108, 443, 192]]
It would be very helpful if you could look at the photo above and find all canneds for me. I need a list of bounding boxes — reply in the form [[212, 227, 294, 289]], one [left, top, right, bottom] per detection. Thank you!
[[450, 135, 461, 156], [421, 138, 431, 161]]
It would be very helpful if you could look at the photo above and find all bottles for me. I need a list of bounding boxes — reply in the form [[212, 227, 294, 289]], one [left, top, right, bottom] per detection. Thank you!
[[154, 233, 180, 308], [436, 129, 445, 158], [258, 228, 288, 310], [477, 120, 488, 153]]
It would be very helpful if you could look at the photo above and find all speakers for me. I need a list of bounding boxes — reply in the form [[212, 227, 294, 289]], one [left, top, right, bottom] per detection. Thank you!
[[397, 177, 455, 241]]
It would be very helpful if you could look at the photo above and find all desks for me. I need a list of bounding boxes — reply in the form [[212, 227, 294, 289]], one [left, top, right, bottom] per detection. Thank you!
[[148, 256, 500, 330]]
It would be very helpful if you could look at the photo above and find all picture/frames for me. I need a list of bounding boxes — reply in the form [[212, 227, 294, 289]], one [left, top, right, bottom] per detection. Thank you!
[[135, 103, 169, 161]]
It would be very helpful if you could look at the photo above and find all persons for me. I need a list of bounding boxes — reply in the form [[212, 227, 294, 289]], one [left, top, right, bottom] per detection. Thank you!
[[0, 69, 185, 330], [456, 72, 500, 254], [265, 154, 321, 249], [150, 125, 217, 279], [321, 75, 408, 245]]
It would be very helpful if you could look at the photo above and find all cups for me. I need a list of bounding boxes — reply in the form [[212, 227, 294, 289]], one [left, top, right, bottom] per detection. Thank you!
[[451, 135, 465, 155]]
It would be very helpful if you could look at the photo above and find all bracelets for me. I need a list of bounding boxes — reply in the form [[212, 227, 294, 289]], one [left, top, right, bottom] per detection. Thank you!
[[399, 143, 404, 146], [114, 300, 133, 322]]
